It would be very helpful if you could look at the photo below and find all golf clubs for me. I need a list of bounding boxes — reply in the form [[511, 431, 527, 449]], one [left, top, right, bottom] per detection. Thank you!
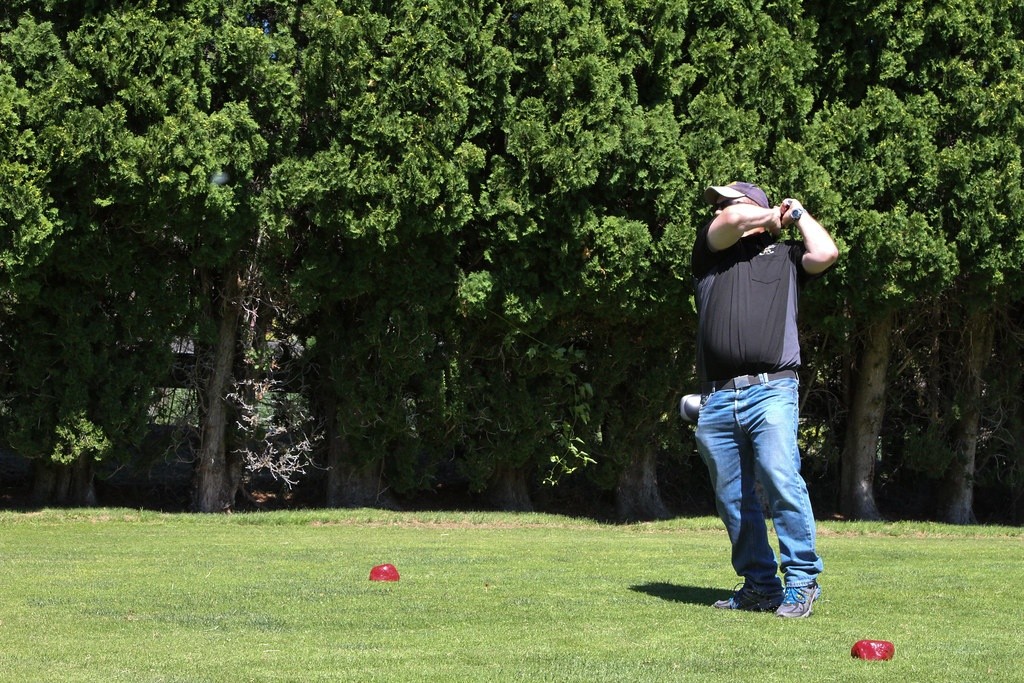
[[680, 208, 804, 422]]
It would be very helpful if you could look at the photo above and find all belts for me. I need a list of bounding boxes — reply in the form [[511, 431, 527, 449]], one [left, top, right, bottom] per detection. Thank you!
[[702, 370, 797, 393]]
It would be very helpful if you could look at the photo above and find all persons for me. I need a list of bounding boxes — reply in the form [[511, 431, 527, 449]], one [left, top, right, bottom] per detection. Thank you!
[[693, 181, 839, 619]]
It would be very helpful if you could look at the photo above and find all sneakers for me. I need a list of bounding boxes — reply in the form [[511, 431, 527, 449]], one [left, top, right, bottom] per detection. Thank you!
[[713, 583, 785, 611], [775, 582, 821, 618]]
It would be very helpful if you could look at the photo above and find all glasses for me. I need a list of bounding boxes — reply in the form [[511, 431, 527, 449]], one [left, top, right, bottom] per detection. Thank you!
[[713, 200, 756, 215]]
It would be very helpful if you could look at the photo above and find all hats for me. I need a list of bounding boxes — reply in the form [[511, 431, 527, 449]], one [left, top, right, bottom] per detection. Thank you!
[[704, 180, 769, 209]]
